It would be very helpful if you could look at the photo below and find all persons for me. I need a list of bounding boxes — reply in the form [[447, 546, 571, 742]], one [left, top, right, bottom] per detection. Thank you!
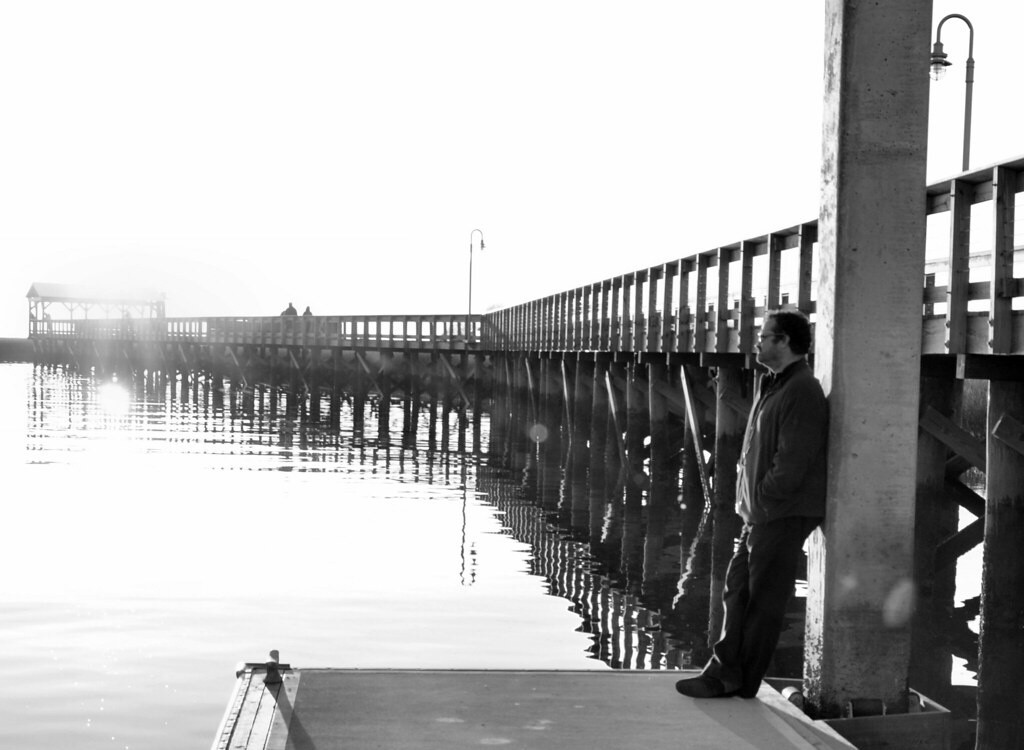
[[284, 303, 299, 316], [303, 306, 312, 315], [672, 306, 829, 704]]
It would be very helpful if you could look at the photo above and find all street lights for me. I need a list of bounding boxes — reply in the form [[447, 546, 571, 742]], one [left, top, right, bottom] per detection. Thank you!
[[930, 14, 974, 173], [467, 229, 486, 344]]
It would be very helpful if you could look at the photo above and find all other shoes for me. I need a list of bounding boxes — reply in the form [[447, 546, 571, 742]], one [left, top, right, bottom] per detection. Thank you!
[[675, 656, 761, 699]]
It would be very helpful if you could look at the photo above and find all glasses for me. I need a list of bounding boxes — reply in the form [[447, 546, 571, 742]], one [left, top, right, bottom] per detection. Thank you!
[[757, 332, 786, 342]]
